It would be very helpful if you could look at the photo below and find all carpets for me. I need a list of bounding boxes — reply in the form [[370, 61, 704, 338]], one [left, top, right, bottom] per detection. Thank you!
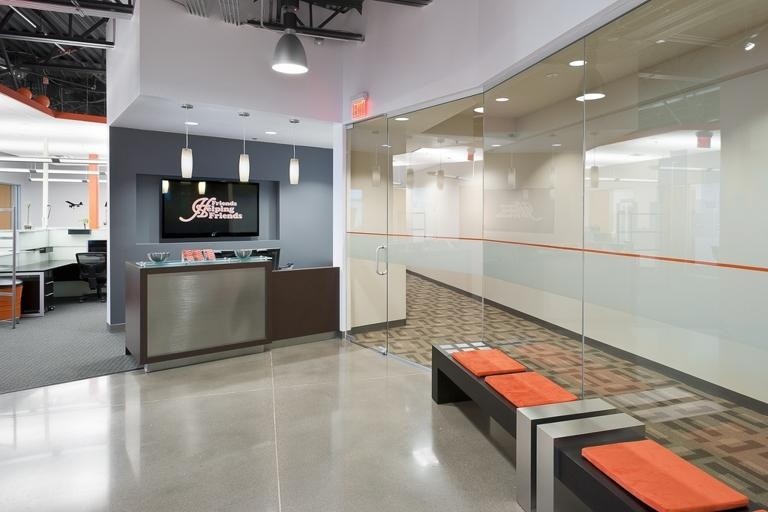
[[0, 280, 144, 395]]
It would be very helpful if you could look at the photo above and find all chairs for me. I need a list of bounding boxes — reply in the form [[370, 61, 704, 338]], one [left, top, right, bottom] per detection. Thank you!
[[76, 251, 107, 303]]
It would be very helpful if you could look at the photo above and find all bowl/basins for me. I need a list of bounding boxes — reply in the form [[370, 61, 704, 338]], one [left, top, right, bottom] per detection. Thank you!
[[147, 252, 170, 263], [233, 248, 254, 261]]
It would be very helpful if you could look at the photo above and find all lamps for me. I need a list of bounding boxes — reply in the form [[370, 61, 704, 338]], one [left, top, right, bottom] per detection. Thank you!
[[288, 118, 301, 185], [179, 104, 195, 179], [237, 111, 252, 183], [271, 1, 310, 76]]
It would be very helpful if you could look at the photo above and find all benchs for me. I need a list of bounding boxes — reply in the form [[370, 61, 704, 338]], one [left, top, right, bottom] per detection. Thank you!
[[430, 339, 619, 512], [534, 411, 752, 512]]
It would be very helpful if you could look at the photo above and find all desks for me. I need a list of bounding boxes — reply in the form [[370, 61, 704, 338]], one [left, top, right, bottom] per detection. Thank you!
[[0, 259, 79, 318], [124, 255, 272, 375]]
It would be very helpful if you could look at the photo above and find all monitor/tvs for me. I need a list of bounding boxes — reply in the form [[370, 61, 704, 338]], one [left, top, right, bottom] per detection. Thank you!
[[161, 179, 259, 238], [258, 248, 280, 269]]
